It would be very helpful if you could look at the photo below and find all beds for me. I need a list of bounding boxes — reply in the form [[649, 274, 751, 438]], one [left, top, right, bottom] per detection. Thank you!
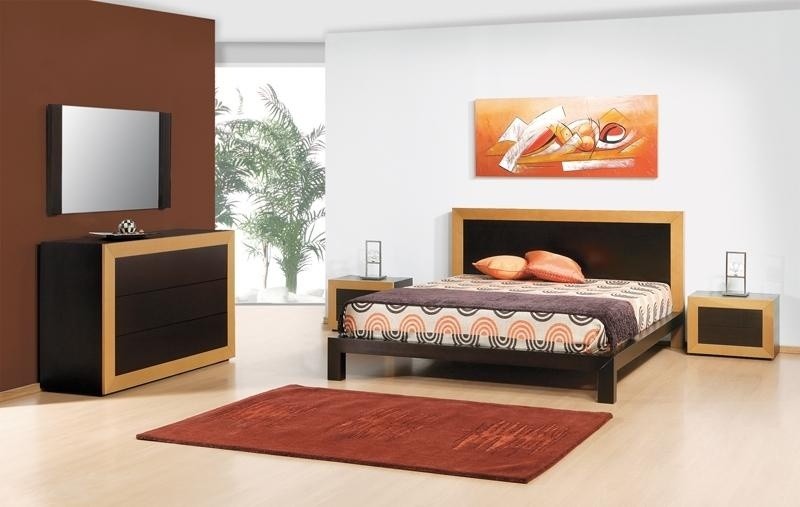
[[328, 208, 685, 404]]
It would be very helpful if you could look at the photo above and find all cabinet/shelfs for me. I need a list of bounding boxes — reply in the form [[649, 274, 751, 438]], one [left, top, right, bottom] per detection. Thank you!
[[40, 228, 235, 396]]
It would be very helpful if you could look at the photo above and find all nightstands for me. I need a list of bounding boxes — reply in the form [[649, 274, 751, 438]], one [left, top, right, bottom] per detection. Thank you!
[[687, 290, 780, 360], [328, 275, 412, 331]]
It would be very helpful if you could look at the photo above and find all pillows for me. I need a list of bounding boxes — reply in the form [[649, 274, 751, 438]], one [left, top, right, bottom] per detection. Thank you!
[[524, 250, 585, 284], [472, 254, 535, 280]]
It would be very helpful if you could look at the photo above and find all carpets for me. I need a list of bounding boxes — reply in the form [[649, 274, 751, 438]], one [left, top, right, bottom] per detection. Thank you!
[[136, 384, 613, 484]]
